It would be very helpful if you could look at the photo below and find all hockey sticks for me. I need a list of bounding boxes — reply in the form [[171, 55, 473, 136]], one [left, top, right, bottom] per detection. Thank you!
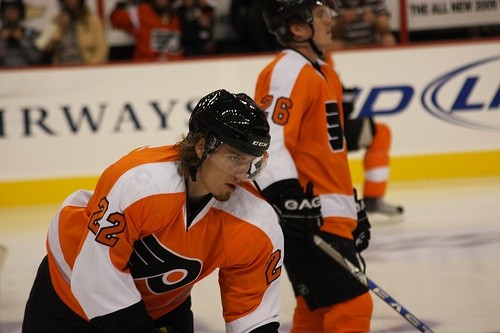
[[312, 236, 433, 333]]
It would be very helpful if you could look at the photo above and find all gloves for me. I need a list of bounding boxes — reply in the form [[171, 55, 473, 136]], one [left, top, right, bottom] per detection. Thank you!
[[349, 187, 372, 251], [263, 179, 323, 253]]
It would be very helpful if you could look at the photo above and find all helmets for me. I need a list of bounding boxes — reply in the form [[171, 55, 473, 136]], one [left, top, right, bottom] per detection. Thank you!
[[190, 91, 270, 158], [263, 0, 323, 32]]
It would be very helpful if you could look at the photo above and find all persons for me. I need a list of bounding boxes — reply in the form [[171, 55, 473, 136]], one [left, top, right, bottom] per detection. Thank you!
[[21, 90, 285, 333], [111, 1, 185, 62], [347, 115, 391, 207], [34, 0, 108, 66], [176, 0, 217, 60], [251, 1, 374, 333], [0, 0, 42, 70], [325, 0, 395, 49]]
